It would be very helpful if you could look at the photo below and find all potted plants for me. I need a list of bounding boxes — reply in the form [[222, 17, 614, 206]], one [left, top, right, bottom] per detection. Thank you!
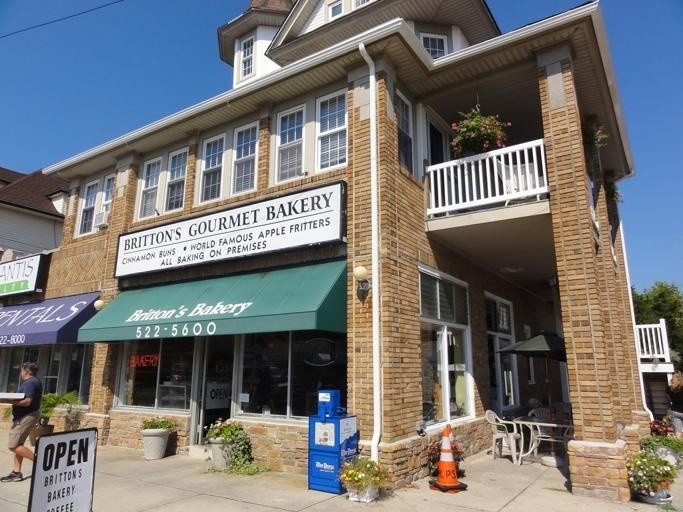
[[140, 416, 175, 460], [651, 420, 676, 436], [641, 435, 683, 469], [207, 423, 268, 475], [4, 390, 81, 446]]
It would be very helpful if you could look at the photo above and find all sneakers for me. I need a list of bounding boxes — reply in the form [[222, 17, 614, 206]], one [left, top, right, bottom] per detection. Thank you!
[[0, 470, 22, 482]]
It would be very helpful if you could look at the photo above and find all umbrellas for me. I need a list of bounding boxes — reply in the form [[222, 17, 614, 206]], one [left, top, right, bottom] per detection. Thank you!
[[495, 329, 567, 421]]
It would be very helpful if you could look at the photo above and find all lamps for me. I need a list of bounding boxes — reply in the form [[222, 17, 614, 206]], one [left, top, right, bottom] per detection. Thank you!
[[354, 267, 368, 290], [94, 299, 105, 313]]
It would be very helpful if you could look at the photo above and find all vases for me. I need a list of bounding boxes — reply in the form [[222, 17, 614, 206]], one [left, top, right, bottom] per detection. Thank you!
[[339, 458, 395, 488], [345, 483, 378, 502], [639, 487, 667, 503], [208, 438, 234, 470], [468, 139, 487, 152]]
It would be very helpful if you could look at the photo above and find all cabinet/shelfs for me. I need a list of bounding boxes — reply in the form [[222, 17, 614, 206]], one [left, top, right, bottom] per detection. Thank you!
[[158, 383, 189, 410]]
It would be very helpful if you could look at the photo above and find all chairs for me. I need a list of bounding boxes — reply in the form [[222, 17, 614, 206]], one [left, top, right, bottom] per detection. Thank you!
[[526, 408, 557, 456], [529, 398, 544, 408], [486, 409, 524, 464], [490, 156, 541, 208]]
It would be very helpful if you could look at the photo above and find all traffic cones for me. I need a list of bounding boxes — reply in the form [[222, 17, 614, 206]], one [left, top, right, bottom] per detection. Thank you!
[[429, 424, 467, 492]]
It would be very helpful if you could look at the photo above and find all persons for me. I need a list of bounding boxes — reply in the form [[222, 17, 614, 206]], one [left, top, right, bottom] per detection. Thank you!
[[0, 360, 43, 483]]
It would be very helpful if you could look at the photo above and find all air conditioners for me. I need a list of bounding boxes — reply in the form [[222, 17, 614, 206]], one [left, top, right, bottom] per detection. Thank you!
[[94, 211, 107, 227]]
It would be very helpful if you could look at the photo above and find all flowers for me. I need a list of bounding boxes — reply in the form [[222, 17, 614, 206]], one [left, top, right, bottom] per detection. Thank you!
[[613, 189, 624, 201], [627, 452, 675, 497], [593, 126, 609, 149], [428, 438, 464, 468], [449, 106, 511, 151]]
